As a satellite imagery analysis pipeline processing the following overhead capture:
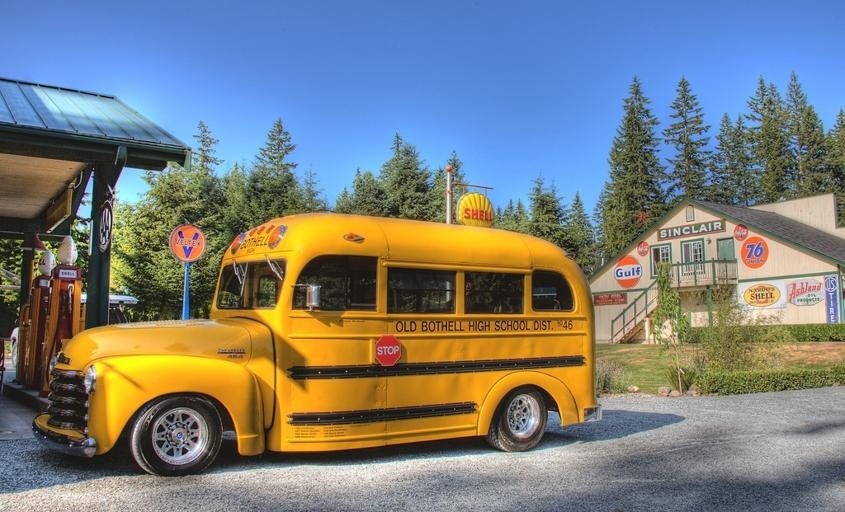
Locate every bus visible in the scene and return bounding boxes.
[32,203,606,478]
